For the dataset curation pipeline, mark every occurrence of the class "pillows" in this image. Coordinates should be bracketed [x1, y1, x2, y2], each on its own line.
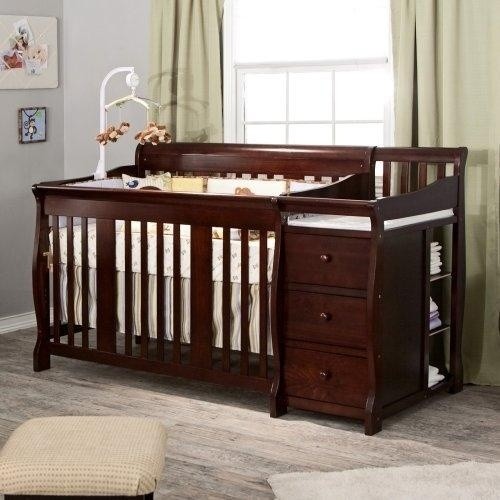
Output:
[204, 176, 286, 242]
[121, 172, 172, 235]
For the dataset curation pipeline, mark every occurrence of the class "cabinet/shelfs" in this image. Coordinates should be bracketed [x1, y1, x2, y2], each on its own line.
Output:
[282, 207, 457, 437]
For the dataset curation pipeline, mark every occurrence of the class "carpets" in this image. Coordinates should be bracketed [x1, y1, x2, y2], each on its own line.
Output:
[267, 463, 499, 500]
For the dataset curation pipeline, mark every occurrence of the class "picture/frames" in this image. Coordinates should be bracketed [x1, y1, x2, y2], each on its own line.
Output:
[18, 106, 46, 144]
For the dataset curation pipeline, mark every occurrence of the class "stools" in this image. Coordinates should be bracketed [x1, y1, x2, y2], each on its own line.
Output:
[0, 415, 166, 499]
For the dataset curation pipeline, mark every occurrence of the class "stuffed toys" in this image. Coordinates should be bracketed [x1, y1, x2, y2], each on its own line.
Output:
[95, 120, 172, 146]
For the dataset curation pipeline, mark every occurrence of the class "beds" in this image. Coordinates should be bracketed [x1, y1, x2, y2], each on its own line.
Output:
[28, 137, 375, 396]
